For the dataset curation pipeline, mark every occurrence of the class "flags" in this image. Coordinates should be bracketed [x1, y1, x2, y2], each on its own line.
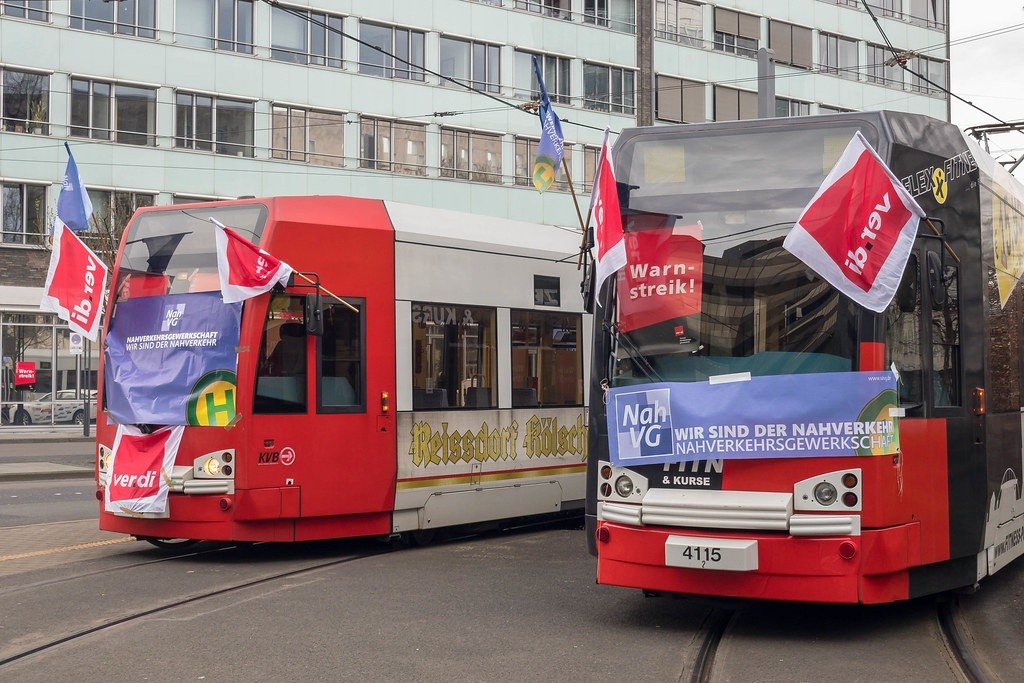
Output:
[214, 220, 292, 304]
[617, 210, 675, 314]
[784, 133, 925, 313]
[50, 154, 93, 234]
[533, 61, 565, 194]
[41, 219, 108, 341]
[594, 142, 627, 307]
[143, 234, 185, 274]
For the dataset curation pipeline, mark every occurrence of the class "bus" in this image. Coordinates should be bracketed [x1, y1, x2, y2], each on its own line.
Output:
[580, 110, 1024, 604]
[95, 195, 615, 550]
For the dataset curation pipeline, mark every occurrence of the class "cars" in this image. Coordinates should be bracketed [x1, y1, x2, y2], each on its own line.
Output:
[8, 388, 97, 426]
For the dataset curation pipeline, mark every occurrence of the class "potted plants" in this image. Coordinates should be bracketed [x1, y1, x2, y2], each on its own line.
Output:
[30, 99, 47, 134]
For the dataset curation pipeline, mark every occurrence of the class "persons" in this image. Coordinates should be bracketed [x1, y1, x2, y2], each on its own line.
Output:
[833, 314, 953, 408]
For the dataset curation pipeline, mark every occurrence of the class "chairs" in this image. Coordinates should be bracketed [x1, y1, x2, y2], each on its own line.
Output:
[254, 320, 543, 413]
